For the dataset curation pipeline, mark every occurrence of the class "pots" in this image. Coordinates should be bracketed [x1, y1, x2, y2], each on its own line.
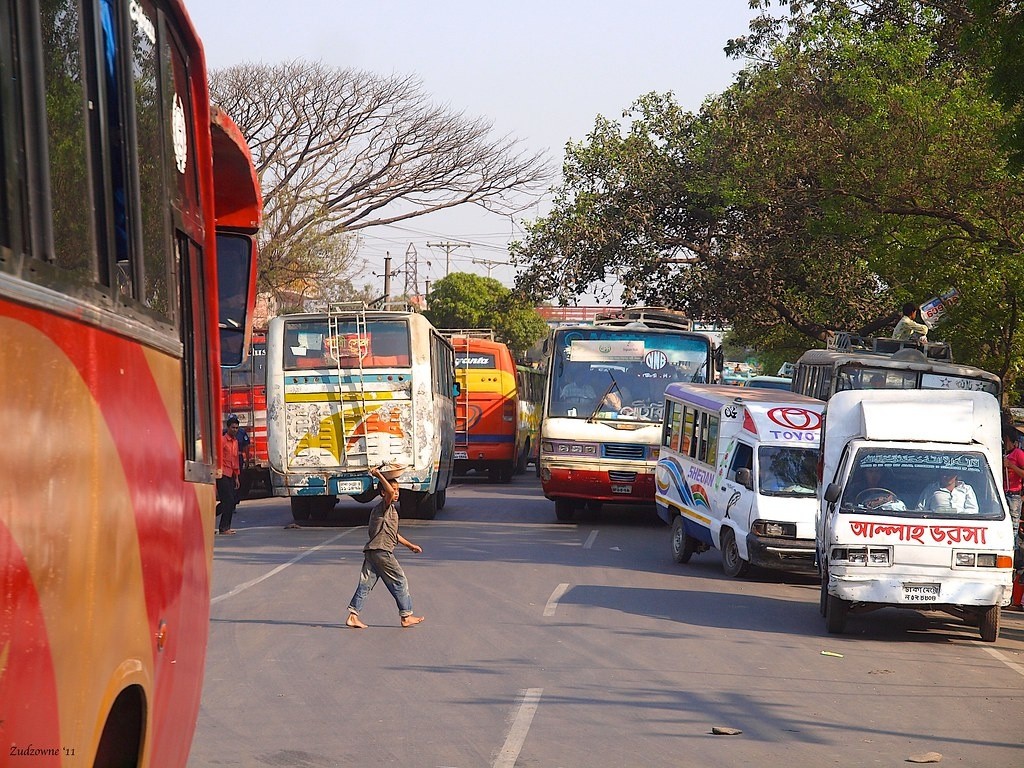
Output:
[371, 460, 407, 480]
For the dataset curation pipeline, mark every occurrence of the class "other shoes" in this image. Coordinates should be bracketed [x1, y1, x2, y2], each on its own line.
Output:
[219, 528, 237, 534]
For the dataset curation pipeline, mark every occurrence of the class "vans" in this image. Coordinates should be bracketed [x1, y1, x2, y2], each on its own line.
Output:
[720, 375, 793, 390]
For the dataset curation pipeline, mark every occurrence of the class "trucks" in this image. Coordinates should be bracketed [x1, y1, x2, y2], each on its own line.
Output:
[655, 382, 827, 578]
[814, 390, 1015, 642]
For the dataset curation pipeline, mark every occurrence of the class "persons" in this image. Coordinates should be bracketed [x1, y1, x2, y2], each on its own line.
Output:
[843, 459, 895, 505]
[891, 303, 929, 343]
[214, 413, 250, 535]
[559, 366, 675, 416]
[747, 451, 792, 490]
[916, 465, 979, 515]
[735, 363, 742, 372]
[346, 465, 425, 628]
[997, 424, 1024, 550]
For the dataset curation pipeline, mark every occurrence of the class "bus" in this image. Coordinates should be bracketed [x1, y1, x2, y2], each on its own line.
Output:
[436, 328, 522, 483]
[267, 301, 461, 522]
[1, 0, 261, 767]
[222, 329, 272, 498]
[541, 306, 725, 521]
[792, 331, 1002, 402]
[513, 357, 546, 477]
